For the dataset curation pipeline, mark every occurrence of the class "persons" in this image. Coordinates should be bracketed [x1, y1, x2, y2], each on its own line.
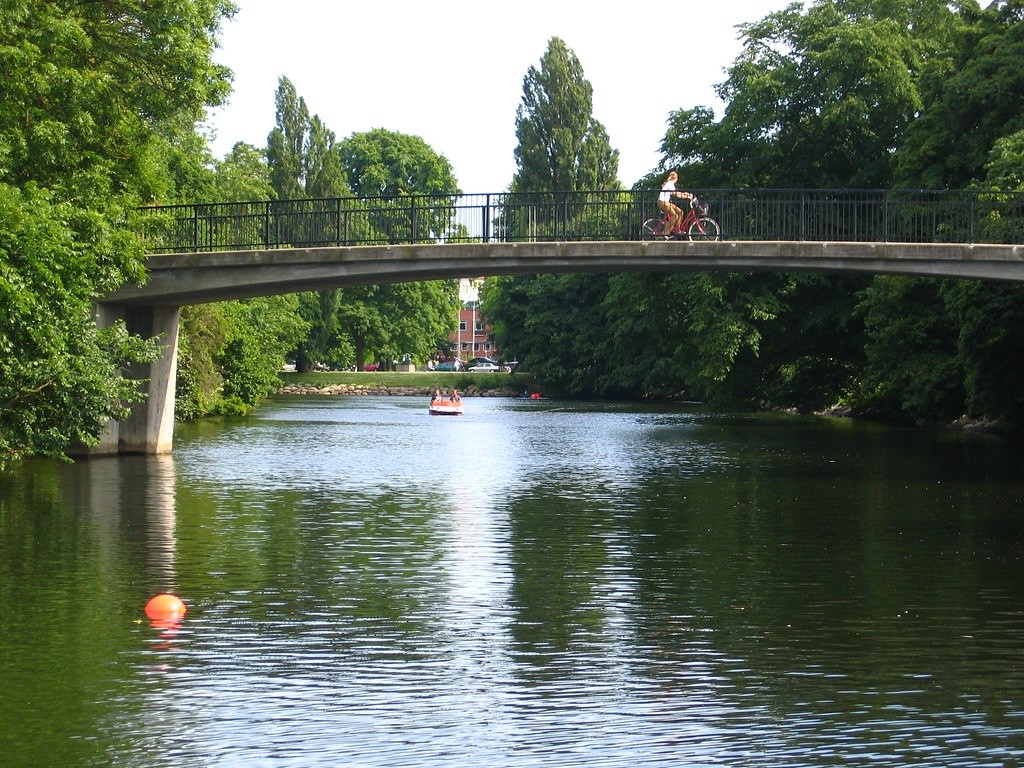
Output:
[657, 171, 693, 241]
[315, 360, 323, 371]
[431, 388, 443, 403]
[450, 388, 461, 402]
[453, 354, 464, 371]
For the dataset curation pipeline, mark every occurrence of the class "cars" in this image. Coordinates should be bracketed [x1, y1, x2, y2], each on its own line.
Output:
[315, 361, 330, 371]
[464, 357, 497, 370]
[283, 359, 296, 371]
[468, 363, 500, 372]
[436, 360, 465, 372]
[330, 363, 345, 371]
[362, 362, 381, 371]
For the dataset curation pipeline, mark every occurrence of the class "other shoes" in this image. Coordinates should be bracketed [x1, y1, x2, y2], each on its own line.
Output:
[662, 232, 669, 240]
[678, 231, 686, 235]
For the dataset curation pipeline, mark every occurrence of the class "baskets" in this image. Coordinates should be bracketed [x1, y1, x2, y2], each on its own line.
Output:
[698, 204, 709, 216]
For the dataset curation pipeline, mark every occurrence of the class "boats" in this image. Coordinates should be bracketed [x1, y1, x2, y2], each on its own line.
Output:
[517, 392, 551, 401]
[428, 395, 463, 415]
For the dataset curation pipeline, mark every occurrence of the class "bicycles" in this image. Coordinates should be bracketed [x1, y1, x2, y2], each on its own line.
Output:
[639, 195, 720, 242]
